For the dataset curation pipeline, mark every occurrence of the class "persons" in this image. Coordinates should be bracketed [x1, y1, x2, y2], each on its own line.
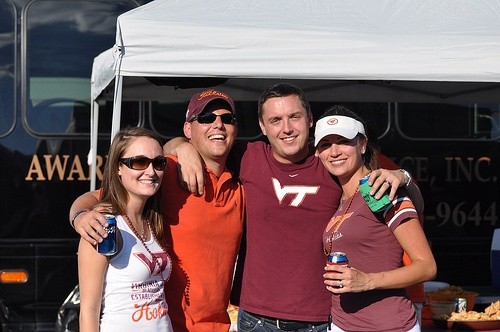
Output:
[163, 82, 424, 332]
[70, 90, 246, 332]
[78, 128, 172, 332]
[314, 107, 437, 332]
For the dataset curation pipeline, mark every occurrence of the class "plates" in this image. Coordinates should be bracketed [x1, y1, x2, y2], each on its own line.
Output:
[446, 318, 500, 329]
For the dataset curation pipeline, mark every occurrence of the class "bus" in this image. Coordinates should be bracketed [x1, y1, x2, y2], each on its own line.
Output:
[0, 1, 500, 332]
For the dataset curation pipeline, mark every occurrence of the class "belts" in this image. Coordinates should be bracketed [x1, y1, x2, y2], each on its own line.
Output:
[250, 314, 324, 330]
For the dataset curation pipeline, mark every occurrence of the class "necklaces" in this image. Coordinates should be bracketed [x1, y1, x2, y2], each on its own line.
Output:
[323, 187, 358, 256]
[338, 197, 348, 212]
[121, 208, 172, 283]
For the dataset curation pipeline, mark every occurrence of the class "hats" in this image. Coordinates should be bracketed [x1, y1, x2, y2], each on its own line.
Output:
[186, 88, 235, 122]
[314, 115, 366, 147]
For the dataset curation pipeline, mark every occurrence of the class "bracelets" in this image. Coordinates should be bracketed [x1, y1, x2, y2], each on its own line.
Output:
[71, 209, 90, 228]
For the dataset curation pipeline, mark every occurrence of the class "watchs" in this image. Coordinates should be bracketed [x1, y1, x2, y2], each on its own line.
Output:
[399, 169, 413, 186]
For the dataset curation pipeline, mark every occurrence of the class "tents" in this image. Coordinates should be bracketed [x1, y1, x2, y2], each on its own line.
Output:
[90, 0, 500, 191]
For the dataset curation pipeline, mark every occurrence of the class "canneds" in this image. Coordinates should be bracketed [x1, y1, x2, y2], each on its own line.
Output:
[328, 251, 348, 264]
[455, 298, 467, 312]
[359, 175, 370, 185]
[96, 214, 116, 256]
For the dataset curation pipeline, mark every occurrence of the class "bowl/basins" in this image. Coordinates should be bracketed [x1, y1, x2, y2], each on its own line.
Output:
[429, 300, 458, 317]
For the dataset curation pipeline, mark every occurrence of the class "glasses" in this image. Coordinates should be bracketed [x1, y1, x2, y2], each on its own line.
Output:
[121, 156, 167, 171]
[191, 112, 236, 124]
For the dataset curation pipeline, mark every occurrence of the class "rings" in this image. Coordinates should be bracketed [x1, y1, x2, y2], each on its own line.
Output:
[339, 281, 343, 287]
[385, 180, 390, 186]
